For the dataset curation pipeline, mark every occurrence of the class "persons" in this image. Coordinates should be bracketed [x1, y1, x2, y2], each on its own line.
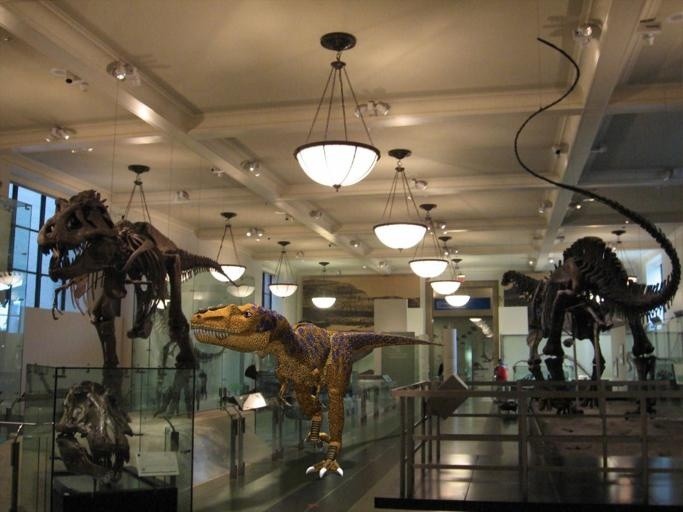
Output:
[494, 359, 507, 392]
[199, 370, 207, 400]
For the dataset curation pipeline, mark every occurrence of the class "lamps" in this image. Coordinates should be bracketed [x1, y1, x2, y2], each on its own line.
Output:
[205, 208, 337, 311]
[290, 33, 381, 194]
[372, 148, 470, 309]
